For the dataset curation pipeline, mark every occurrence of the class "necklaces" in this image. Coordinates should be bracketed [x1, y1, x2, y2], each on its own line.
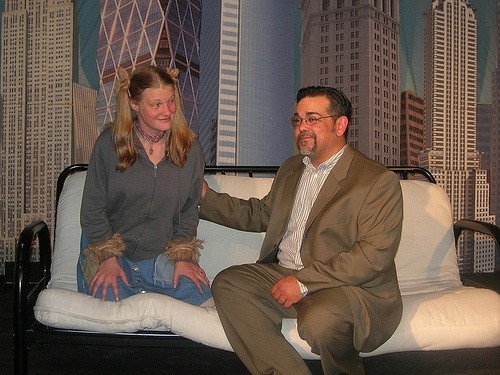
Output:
[136, 122, 166, 154]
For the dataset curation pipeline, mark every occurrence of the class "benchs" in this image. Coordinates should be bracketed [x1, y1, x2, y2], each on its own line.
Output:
[13, 164, 500, 375]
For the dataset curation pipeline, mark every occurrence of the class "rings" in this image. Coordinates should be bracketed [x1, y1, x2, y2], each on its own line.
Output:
[200, 272, 203, 275]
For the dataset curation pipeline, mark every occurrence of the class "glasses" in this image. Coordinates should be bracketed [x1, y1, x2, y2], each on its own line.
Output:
[291, 115, 341, 126]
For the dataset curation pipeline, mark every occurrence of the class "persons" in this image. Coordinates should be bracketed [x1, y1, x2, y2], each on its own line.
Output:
[200, 86, 404, 375]
[77, 65, 213, 306]
